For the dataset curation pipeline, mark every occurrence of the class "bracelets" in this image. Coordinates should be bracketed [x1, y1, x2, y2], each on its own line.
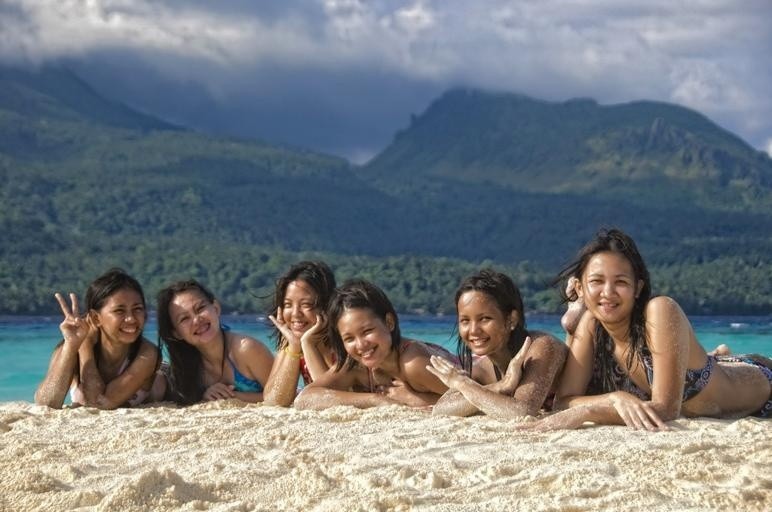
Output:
[284, 346, 303, 360]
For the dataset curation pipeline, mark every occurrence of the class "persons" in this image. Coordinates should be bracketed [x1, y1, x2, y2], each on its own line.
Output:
[157, 280, 301, 404]
[33, 267, 169, 410]
[519, 225, 772, 432]
[264, 259, 348, 408]
[295, 278, 480, 409]
[426, 268, 587, 422]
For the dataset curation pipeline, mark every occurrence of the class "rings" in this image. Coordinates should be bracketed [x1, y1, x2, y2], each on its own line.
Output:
[441, 369, 448, 373]
